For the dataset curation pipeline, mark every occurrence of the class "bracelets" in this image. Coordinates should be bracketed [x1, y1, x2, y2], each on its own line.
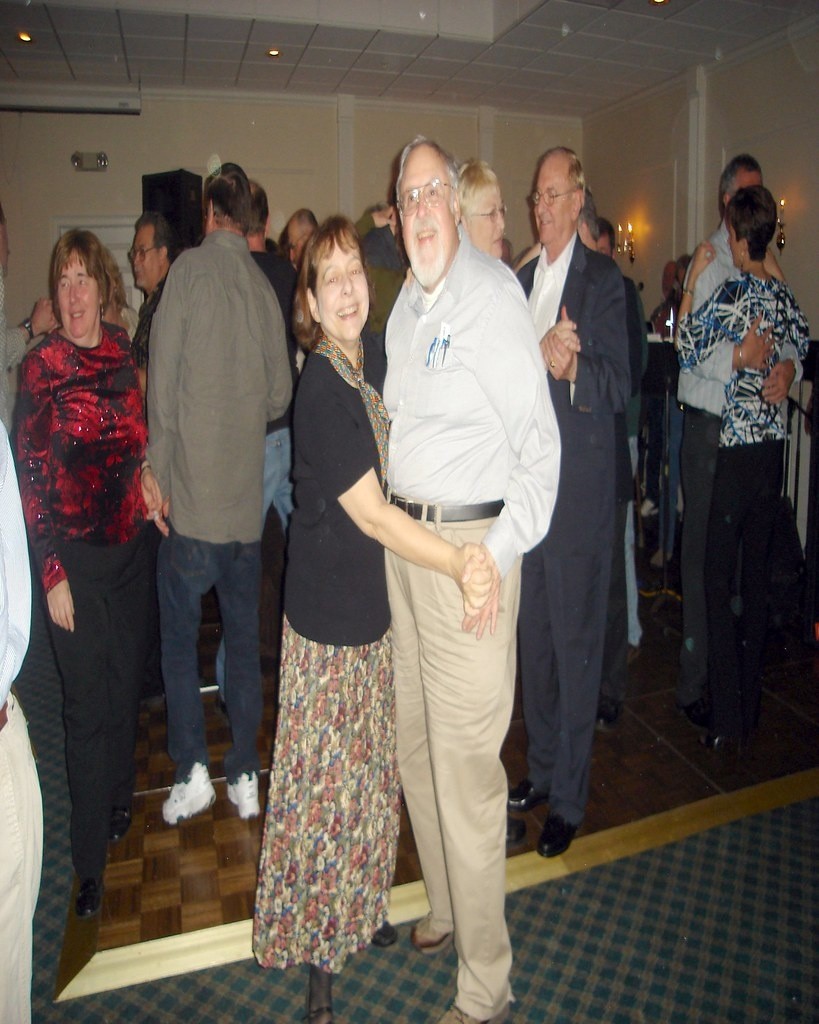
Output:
[142, 465, 151, 472]
[682, 289, 694, 296]
[738, 344, 743, 369]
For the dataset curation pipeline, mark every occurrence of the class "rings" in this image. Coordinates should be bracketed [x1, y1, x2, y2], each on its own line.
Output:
[550, 362, 556, 368]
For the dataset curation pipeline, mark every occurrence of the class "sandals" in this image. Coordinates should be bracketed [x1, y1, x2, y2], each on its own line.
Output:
[696, 732, 725, 750]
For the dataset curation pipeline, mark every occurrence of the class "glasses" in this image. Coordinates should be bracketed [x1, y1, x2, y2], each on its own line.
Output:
[288, 232, 308, 249]
[531, 189, 577, 206]
[395, 178, 455, 217]
[128, 245, 160, 262]
[470, 204, 507, 223]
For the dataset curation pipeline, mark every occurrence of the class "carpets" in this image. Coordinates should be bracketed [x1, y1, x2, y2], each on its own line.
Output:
[11, 560, 819, 1024]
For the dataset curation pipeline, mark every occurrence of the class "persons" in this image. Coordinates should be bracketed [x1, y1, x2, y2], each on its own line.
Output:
[15, 153, 810, 917]
[254, 213, 493, 1024]
[0, 204, 58, 1024]
[455, 156, 582, 846]
[376, 134, 560, 1024]
[507, 146, 632, 857]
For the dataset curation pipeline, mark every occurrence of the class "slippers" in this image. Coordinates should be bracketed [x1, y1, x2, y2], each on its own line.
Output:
[304, 985, 333, 1024]
[372, 920, 399, 947]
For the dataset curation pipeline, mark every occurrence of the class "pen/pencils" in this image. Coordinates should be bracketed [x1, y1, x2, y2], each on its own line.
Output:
[426, 337, 442, 367]
[441, 335, 451, 365]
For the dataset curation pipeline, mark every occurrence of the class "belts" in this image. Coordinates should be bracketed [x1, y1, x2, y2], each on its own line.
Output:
[383, 478, 505, 524]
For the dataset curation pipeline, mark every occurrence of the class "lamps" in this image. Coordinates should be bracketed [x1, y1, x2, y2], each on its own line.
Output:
[616, 222, 636, 264]
[72, 151, 109, 173]
[776, 197, 787, 256]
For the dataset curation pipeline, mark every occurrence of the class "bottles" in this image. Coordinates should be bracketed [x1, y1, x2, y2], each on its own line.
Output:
[664, 303, 677, 342]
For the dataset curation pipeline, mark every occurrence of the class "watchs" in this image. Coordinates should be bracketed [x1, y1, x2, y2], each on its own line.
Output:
[23, 320, 34, 339]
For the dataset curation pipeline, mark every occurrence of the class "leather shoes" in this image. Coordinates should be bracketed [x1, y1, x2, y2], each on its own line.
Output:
[595, 696, 625, 729]
[506, 814, 527, 844]
[506, 778, 551, 811]
[675, 695, 713, 728]
[109, 800, 132, 843]
[74, 873, 105, 918]
[537, 812, 578, 858]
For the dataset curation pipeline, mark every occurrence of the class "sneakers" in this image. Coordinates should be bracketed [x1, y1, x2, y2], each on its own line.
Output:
[226, 769, 260, 820]
[161, 761, 216, 826]
[438, 999, 510, 1024]
[667, 570, 684, 601]
[638, 561, 667, 597]
[411, 910, 455, 955]
